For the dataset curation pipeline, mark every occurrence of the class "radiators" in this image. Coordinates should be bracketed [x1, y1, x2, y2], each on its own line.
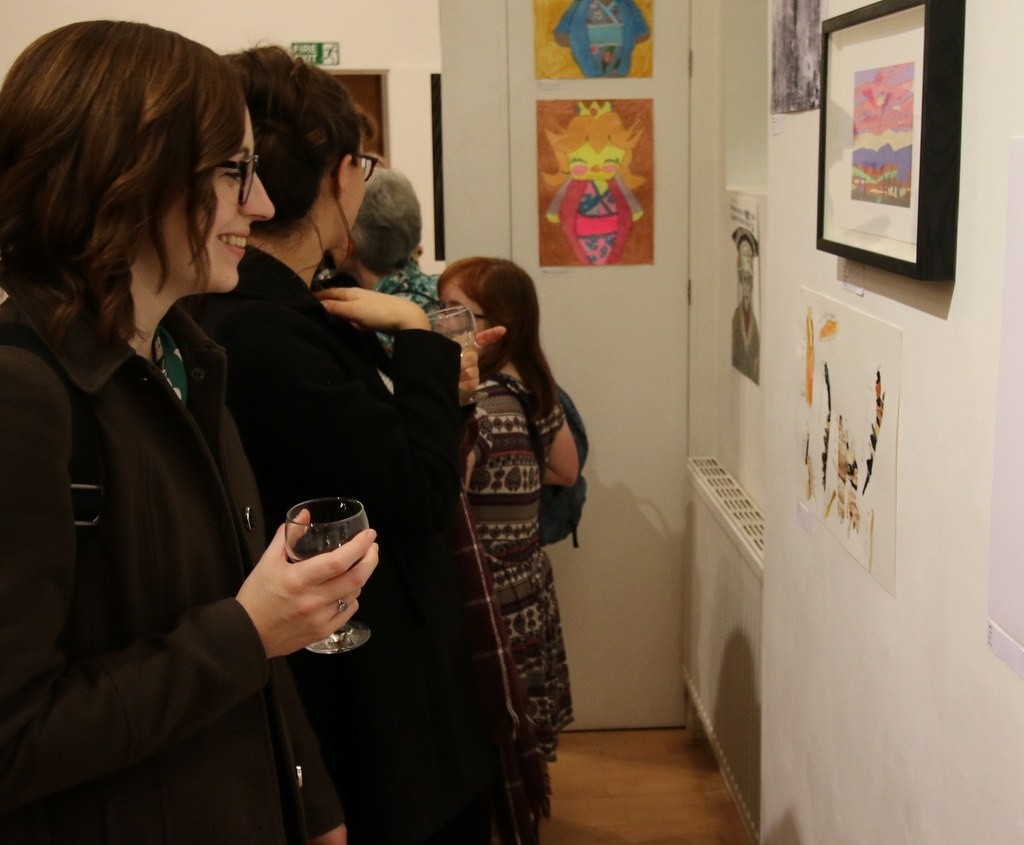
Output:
[684, 456, 764, 845]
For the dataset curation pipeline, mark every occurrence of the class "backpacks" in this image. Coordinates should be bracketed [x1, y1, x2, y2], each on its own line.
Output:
[488, 373, 589, 548]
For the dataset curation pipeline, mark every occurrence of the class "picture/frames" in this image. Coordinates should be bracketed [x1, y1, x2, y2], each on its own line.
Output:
[817, 0, 965, 279]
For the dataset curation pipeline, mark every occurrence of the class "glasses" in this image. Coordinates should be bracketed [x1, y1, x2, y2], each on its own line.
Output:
[213, 154, 260, 206]
[436, 303, 488, 320]
[357, 153, 379, 182]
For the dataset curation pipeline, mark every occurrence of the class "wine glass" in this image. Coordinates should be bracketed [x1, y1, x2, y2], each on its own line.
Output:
[284, 495, 371, 655]
[427, 304, 489, 407]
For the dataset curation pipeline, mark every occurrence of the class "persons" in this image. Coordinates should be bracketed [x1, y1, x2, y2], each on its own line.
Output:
[182, 46, 508, 845]
[324, 168, 581, 832]
[731, 227, 760, 386]
[0, 21, 380, 845]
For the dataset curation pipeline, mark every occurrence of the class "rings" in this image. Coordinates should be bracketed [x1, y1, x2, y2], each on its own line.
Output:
[469, 331, 483, 350]
[338, 599, 348, 612]
